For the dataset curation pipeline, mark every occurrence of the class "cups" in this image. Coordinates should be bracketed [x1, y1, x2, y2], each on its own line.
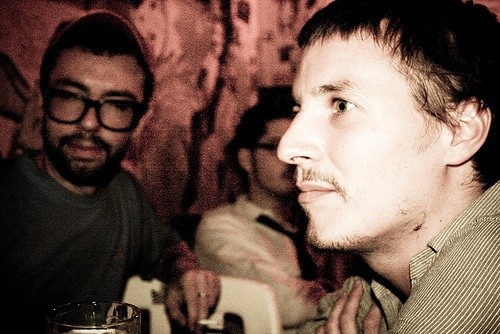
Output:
[47, 300, 141, 334]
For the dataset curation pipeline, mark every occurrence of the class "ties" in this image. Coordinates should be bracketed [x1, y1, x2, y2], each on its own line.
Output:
[257, 216, 320, 282]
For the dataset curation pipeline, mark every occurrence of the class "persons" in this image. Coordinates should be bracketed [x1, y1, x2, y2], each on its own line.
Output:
[277, 0, 500, 334]
[0, 8, 356, 334]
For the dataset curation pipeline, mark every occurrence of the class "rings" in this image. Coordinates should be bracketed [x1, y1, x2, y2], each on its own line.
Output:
[197, 291, 210, 300]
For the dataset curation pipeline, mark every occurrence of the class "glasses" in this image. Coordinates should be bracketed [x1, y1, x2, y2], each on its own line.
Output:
[256, 141, 279, 156]
[44, 88, 141, 133]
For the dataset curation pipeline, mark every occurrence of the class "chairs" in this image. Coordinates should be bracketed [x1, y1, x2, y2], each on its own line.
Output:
[123, 271, 284, 334]
